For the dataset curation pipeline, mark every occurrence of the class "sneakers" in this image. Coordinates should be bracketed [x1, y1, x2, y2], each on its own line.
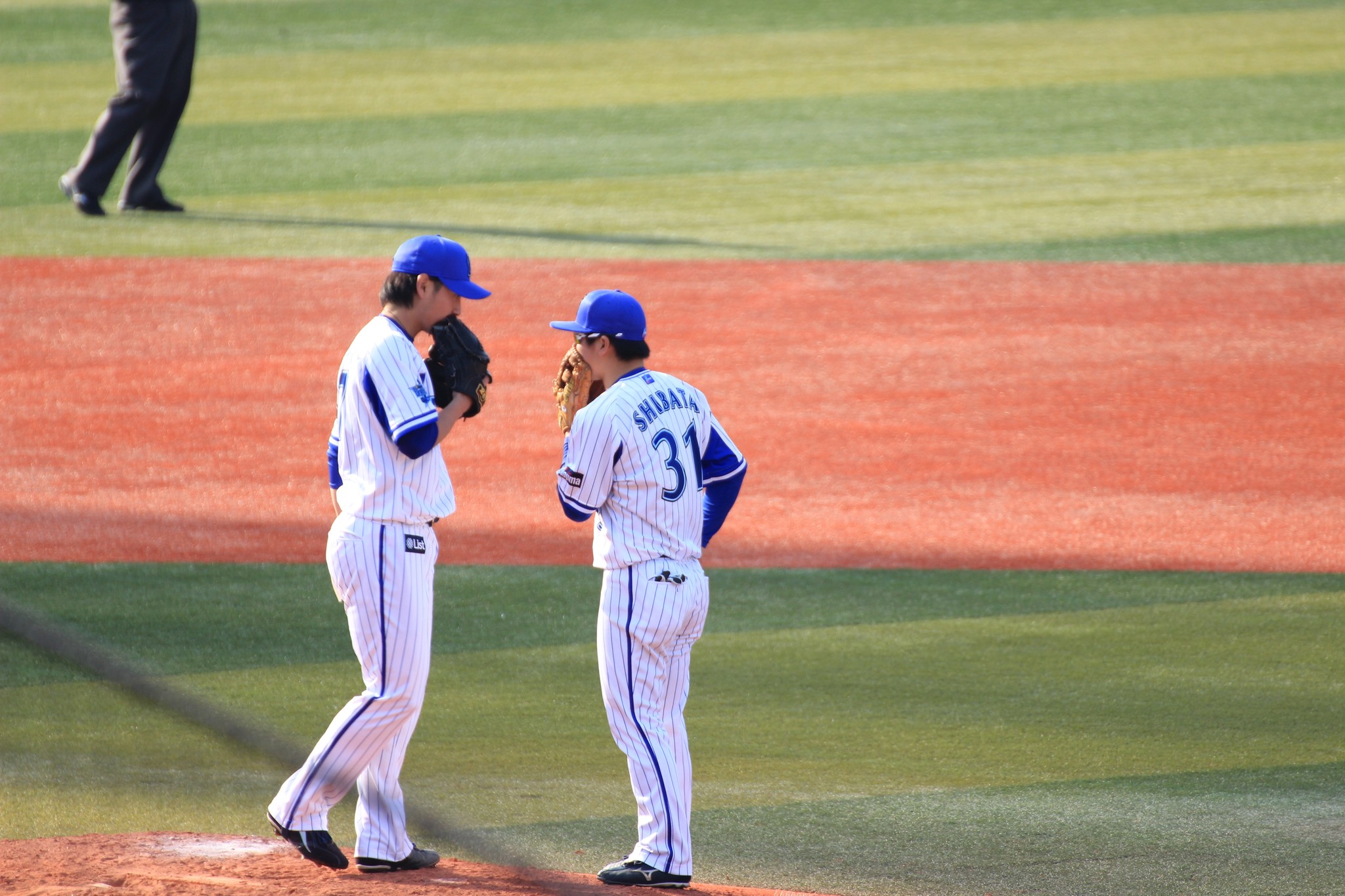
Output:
[356, 843, 442, 872]
[266, 811, 349, 871]
[598, 855, 691, 887]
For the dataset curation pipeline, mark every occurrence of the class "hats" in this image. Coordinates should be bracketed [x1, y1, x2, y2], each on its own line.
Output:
[549, 289, 648, 340]
[392, 233, 493, 299]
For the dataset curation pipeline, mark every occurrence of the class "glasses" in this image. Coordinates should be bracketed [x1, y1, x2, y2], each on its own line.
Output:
[574, 333, 600, 343]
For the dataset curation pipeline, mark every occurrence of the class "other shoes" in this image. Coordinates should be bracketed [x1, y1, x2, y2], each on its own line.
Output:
[58, 172, 105, 215]
[119, 194, 183, 212]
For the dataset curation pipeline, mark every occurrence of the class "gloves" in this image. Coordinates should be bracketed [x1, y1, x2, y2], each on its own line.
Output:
[655, 570, 687, 584]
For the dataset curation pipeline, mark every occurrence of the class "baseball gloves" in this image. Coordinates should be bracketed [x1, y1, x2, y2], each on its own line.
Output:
[552, 341, 606, 434]
[423, 312, 492, 418]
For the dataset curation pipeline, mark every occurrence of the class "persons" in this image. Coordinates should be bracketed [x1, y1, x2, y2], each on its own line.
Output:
[56, 0, 201, 216]
[266, 235, 492, 872]
[549, 290, 749, 888]
